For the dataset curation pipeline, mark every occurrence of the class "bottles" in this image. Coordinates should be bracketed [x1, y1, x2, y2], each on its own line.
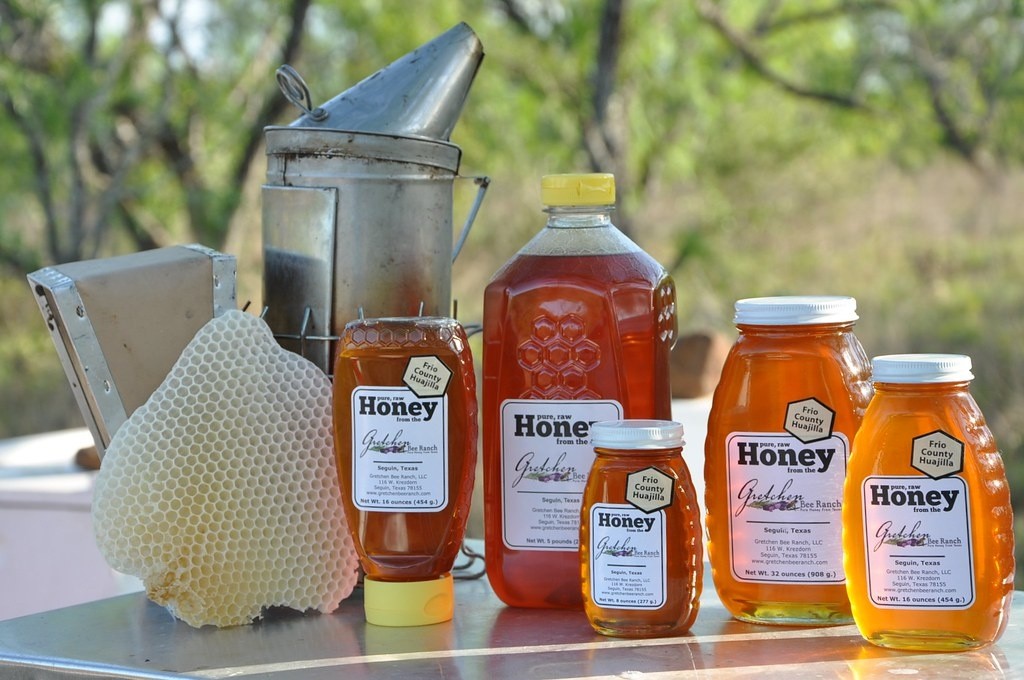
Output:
[704, 297, 881, 624]
[325, 317, 476, 626]
[576, 418, 705, 639]
[479, 175, 678, 602]
[840, 350, 1016, 654]
